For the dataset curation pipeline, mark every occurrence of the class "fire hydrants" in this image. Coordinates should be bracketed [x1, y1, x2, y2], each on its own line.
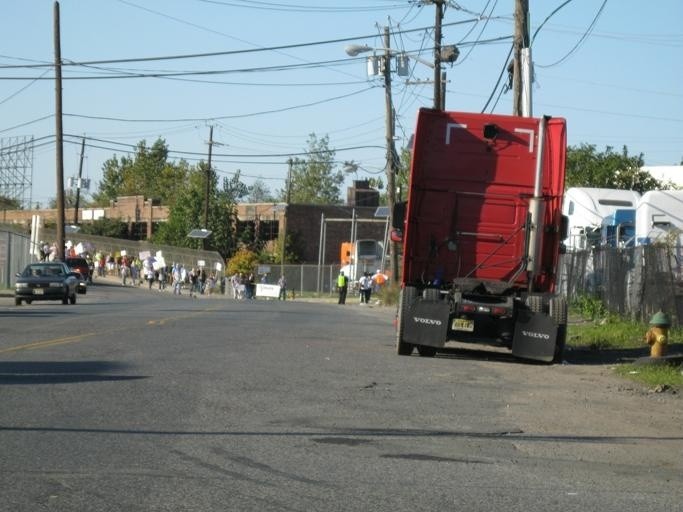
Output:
[643, 306, 672, 357]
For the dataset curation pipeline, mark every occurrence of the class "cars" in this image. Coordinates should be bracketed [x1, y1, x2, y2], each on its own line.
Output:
[13, 261, 79, 305]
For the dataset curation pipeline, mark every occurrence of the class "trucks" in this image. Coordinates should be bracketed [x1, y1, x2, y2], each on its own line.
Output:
[395, 107, 571, 366]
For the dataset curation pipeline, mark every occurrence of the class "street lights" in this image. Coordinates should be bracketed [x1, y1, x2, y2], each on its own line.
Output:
[345, 0, 460, 111]
[342, 162, 387, 177]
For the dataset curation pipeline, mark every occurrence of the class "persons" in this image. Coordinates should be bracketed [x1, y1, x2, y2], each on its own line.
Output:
[261, 273, 268, 284]
[278, 274, 287, 300]
[335, 271, 348, 304]
[39, 242, 254, 300]
[358, 269, 389, 306]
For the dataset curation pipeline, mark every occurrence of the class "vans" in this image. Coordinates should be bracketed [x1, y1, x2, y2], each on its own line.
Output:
[66, 256, 91, 284]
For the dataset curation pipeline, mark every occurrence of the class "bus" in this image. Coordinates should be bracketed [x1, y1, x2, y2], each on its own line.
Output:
[353, 240, 385, 287]
